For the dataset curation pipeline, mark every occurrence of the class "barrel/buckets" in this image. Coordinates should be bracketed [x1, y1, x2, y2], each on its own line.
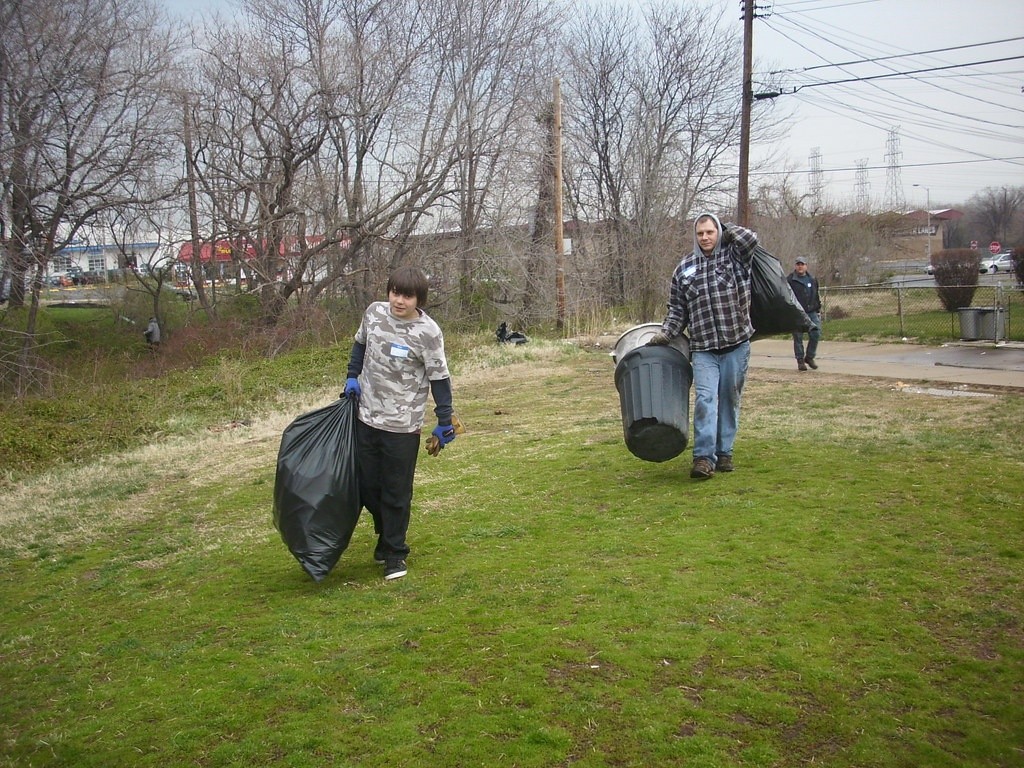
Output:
[613, 322, 690, 366]
[614, 344, 696, 461]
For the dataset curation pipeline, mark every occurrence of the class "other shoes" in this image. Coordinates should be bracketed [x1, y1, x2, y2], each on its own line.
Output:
[798, 358, 807, 371]
[805, 356, 817, 369]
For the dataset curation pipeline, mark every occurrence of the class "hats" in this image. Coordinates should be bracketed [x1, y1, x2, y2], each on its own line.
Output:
[796, 257, 806, 264]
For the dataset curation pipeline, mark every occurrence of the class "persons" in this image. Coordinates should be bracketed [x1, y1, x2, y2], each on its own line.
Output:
[650, 213, 759, 477]
[143, 316, 161, 346]
[344, 265, 455, 581]
[785, 257, 820, 369]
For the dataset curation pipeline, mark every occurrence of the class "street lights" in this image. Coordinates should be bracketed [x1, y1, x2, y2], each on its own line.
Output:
[913, 183, 931, 265]
[1002, 186, 1005, 253]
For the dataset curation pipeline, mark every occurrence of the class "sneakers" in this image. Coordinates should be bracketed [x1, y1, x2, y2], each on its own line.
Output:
[690, 459, 714, 478]
[717, 455, 734, 471]
[374, 544, 387, 564]
[384, 558, 407, 581]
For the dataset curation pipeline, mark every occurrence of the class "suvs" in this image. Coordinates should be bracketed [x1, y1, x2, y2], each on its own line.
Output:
[978, 253, 1014, 274]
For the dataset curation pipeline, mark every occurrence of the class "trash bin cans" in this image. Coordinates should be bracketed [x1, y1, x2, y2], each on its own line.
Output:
[957, 307, 981, 339]
[981, 307, 1006, 340]
[613, 322, 693, 463]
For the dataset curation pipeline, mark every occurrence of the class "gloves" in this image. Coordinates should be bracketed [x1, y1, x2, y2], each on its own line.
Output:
[645, 332, 670, 345]
[426, 415, 466, 457]
[432, 425, 455, 449]
[344, 378, 361, 398]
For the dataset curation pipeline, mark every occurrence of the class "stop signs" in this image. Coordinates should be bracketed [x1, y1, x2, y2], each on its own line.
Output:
[971, 241, 977, 249]
[989, 242, 1001, 254]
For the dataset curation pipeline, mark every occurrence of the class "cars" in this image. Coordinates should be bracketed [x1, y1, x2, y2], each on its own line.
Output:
[84, 266, 185, 283]
[3, 267, 85, 300]
[1005, 248, 1014, 253]
[924, 264, 936, 274]
